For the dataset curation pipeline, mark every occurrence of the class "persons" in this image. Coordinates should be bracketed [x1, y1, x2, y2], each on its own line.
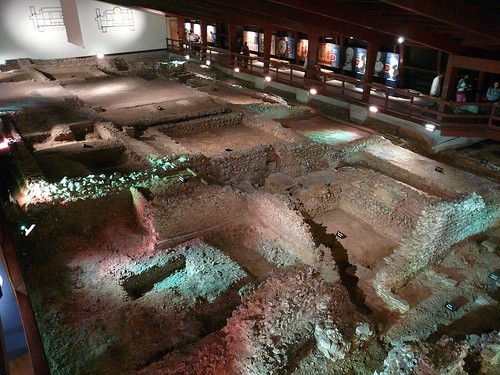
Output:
[453, 72, 473, 113]
[210, 31, 216, 42]
[177, 28, 200, 55]
[487, 81, 500, 116]
[428, 70, 444, 110]
[243, 41, 250, 68]
